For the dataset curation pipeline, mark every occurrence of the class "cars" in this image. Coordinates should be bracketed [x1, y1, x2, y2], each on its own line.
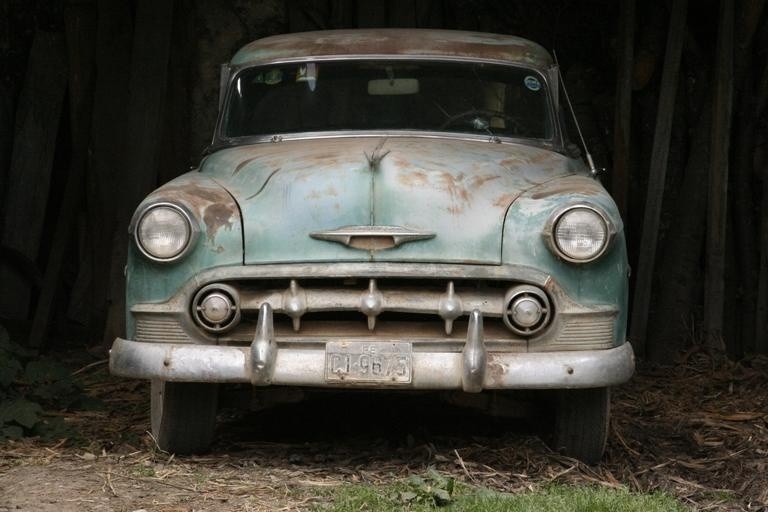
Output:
[109, 25, 637, 466]
